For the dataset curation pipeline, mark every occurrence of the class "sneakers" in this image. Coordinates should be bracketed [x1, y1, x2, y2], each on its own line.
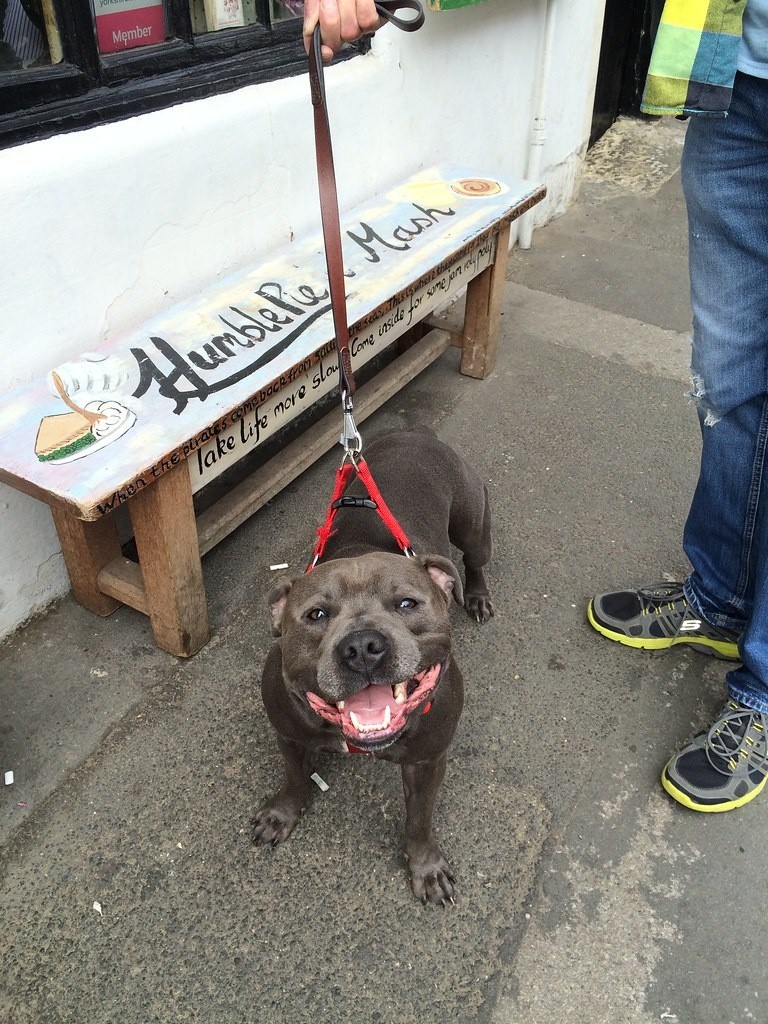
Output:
[588, 582, 742, 661]
[661, 695, 768, 813]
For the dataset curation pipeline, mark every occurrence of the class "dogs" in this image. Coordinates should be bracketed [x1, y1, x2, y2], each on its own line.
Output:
[247, 432, 491, 911]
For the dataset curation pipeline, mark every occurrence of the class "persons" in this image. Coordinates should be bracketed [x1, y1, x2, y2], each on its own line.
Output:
[303, 0, 768, 813]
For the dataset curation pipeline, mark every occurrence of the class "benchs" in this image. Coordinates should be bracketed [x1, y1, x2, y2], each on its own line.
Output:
[0, 155, 547, 656]
